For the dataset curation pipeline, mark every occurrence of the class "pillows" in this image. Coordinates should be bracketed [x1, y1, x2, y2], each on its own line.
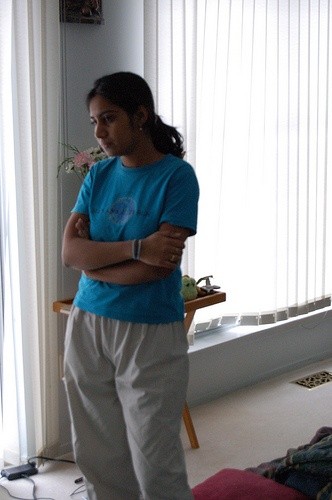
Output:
[192, 468, 307, 500]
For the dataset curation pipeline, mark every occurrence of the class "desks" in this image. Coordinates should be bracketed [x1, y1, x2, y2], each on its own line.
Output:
[52, 286, 227, 451]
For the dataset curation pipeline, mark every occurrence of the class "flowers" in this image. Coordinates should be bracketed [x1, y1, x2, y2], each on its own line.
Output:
[55, 141, 108, 183]
